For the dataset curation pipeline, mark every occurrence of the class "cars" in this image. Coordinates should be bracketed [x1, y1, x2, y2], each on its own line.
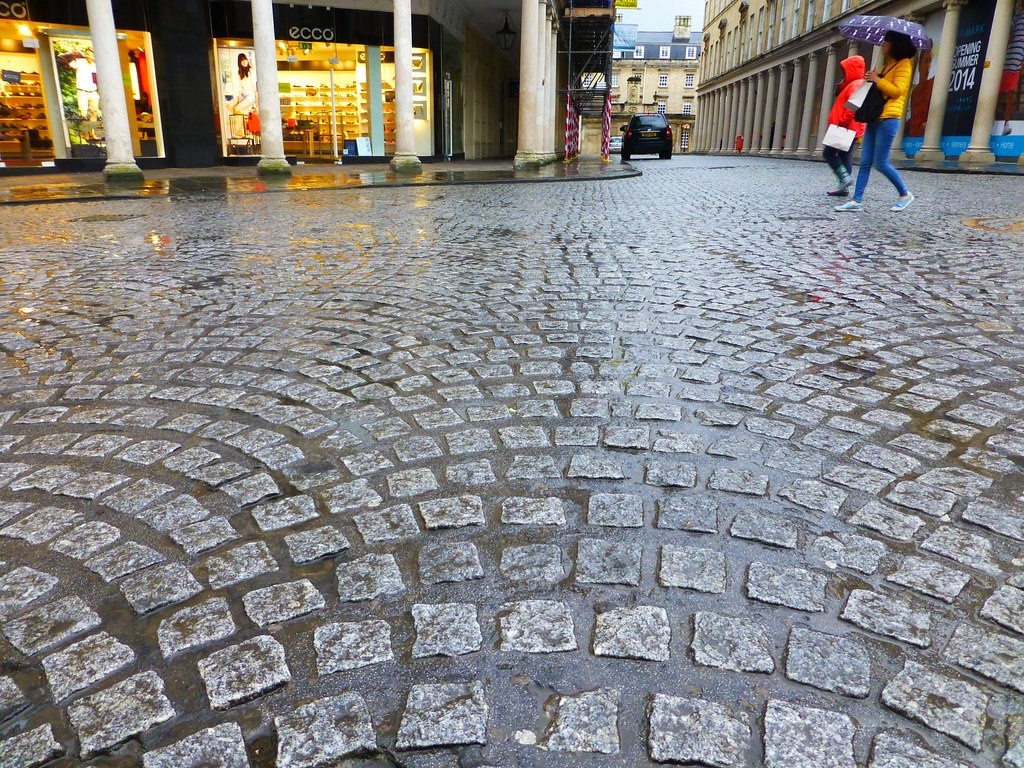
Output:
[609, 137, 622, 154]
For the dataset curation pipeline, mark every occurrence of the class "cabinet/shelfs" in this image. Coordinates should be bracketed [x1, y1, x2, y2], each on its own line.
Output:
[0, 71, 55, 160]
[292, 82, 396, 156]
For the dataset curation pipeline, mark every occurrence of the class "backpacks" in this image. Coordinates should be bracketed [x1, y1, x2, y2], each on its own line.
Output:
[854, 74, 884, 122]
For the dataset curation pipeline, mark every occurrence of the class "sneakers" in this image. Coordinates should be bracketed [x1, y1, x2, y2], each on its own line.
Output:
[834, 202, 864, 212]
[890, 191, 914, 211]
[826, 189, 849, 195]
[842, 177, 854, 188]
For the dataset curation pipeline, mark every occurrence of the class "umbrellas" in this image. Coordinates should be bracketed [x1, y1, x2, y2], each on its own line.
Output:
[838, 15, 930, 82]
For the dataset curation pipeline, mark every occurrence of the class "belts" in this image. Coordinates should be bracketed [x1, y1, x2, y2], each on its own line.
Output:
[78, 88, 97, 93]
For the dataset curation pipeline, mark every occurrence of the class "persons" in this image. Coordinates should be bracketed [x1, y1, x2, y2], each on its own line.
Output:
[822, 55, 866, 196]
[226, 53, 257, 116]
[68, 47, 101, 140]
[907, 39, 935, 137]
[834, 30, 916, 211]
[989, 0, 1024, 136]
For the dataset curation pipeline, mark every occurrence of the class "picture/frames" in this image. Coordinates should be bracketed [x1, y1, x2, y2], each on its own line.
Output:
[412, 77, 426, 95]
[414, 100, 428, 119]
[412, 52, 426, 71]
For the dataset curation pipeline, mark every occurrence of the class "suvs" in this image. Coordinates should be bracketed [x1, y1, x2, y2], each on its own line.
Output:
[619, 112, 673, 160]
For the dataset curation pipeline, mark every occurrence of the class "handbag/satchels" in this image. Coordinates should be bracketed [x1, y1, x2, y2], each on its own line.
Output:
[845, 80, 872, 111]
[822, 122, 857, 152]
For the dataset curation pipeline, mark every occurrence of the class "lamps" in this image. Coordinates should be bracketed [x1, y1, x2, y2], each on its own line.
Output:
[495, 9, 516, 50]
